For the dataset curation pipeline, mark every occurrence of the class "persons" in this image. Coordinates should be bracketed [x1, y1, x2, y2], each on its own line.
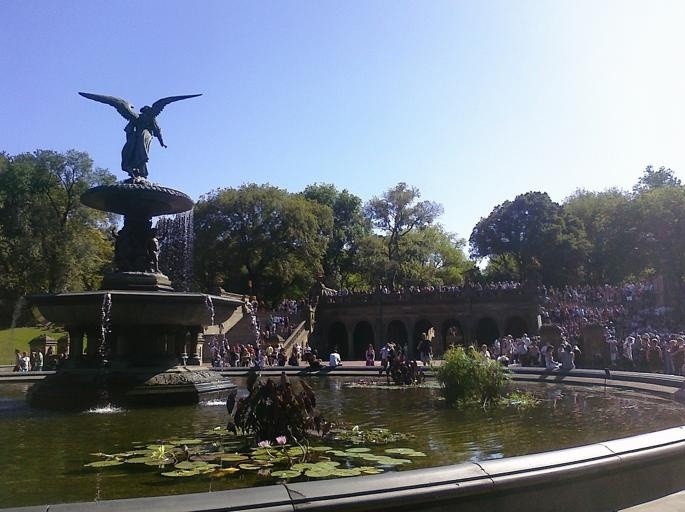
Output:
[415, 330, 436, 366]
[120, 106, 167, 178]
[13, 345, 68, 371]
[365, 340, 408, 366]
[208, 289, 345, 368]
[465, 279, 685, 377]
[343, 284, 459, 293]
